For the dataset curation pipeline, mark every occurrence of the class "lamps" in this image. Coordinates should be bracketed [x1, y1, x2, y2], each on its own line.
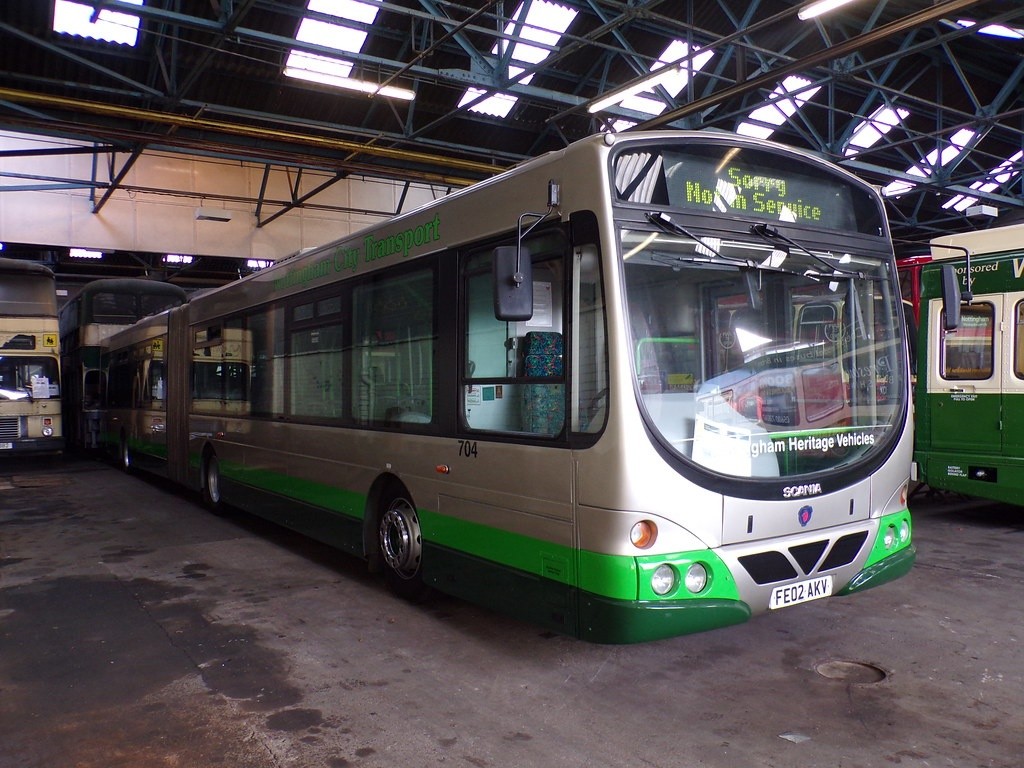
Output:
[195, 198, 232, 222]
[964, 200, 998, 218]
[585, 63, 680, 114]
[797, 0, 855, 21]
[280, 67, 417, 101]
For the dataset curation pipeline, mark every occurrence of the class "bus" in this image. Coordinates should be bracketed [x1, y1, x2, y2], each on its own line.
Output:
[714, 219, 1023, 506]
[58, 277, 189, 440]
[105, 129, 972, 646]
[101, 327, 254, 441]
[0, 257, 65, 455]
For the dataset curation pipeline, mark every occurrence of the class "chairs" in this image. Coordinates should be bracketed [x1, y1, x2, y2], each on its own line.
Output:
[519, 330, 584, 431]
